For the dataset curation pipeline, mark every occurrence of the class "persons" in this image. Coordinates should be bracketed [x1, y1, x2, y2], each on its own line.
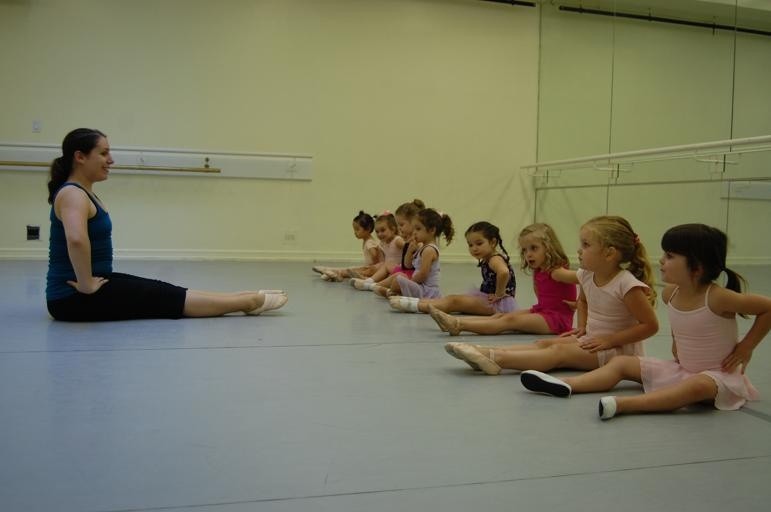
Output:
[429, 223, 582, 336]
[310, 198, 457, 302]
[444, 212, 661, 376]
[45, 126, 289, 318]
[521, 221, 771, 421]
[390, 220, 518, 318]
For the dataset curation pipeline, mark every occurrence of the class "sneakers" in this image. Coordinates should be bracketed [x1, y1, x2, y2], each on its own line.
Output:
[245, 290, 288, 315]
[598, 395, 617, 420]
[350, 276, 419, 312]
[427, 302, 461, 336]
[312, 266, 342, 282]
[444, 342, 502, 375]
[520, 369, 572, 398]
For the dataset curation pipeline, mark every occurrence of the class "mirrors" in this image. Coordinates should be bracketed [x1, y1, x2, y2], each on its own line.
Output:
[536, 0, 771, 267]
